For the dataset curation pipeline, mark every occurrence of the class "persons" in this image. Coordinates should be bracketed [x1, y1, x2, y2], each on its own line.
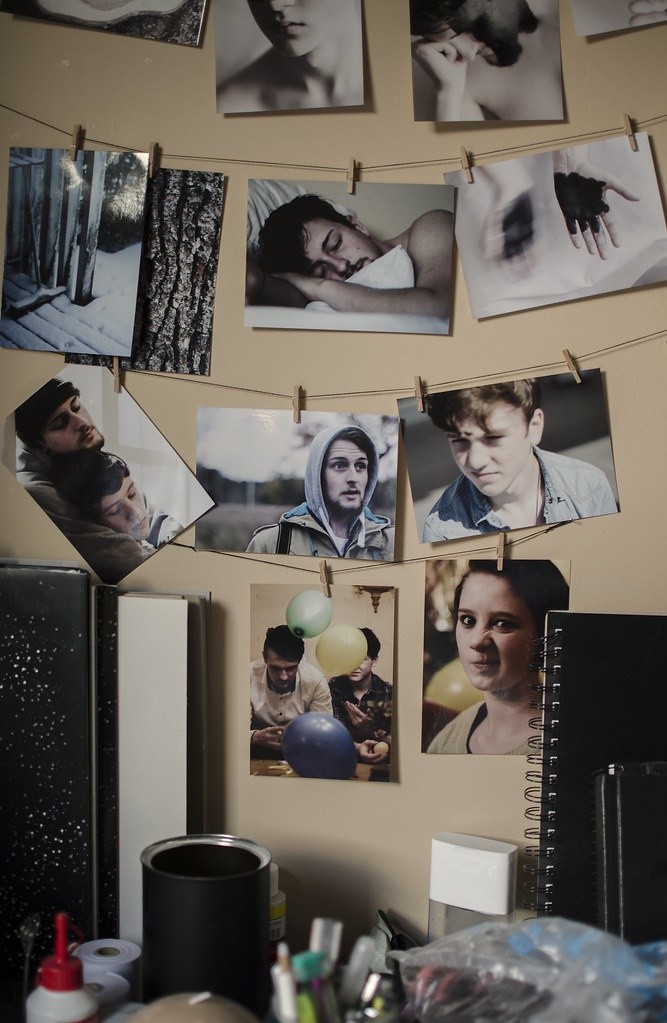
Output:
[241, 192, 452, 333]
[248, 620, 393, 764]
[624, 2, 666, 27]
[459, 143, 647, 292]
[422, 560, 571, 760]
[214, 0, 364, 115]
[415, 376, 621, 545]
[401, 0, 567, 126]
[6, 376, 187, 587]
[247, 421, 396, 567]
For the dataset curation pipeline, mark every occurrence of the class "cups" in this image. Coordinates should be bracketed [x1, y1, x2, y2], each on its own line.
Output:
[139, 833, 272, 1019]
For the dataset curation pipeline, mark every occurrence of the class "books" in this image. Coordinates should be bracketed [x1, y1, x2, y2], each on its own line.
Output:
[527, 607, 666, 956]
[0, 566, 214, 1023]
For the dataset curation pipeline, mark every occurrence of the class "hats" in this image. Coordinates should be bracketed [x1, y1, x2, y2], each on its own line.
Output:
[16, 376, 81, 447]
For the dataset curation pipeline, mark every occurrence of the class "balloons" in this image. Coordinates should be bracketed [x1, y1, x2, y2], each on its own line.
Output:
[285, 589, 332, 638]
[281, 712, 357, 781]
[315, 624, 368, 674]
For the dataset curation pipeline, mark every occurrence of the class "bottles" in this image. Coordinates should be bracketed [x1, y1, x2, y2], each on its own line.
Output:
[269, 863, 288, 969]
[25, 912, 100, 1023]
